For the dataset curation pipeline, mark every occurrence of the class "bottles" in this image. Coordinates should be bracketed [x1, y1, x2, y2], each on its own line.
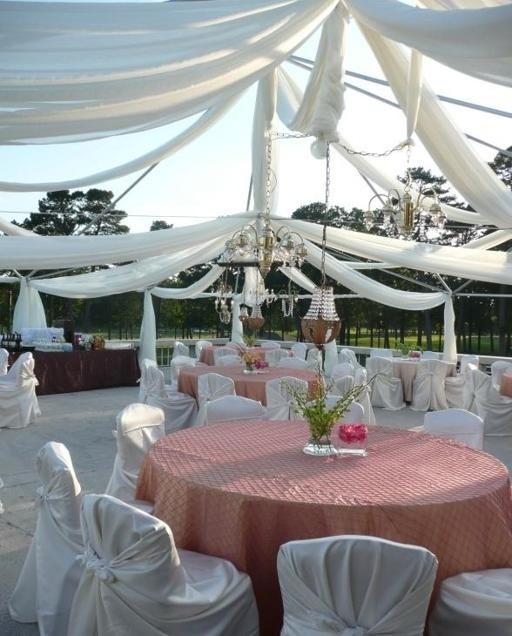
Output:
[1, 332, 22, 353]
[76, 335, 106, 351]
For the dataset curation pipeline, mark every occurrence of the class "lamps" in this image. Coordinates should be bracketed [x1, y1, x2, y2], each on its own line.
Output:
[363, 143, 447, 245]
[301, 137, 344, 349]
[205, 130, 311, 330]
